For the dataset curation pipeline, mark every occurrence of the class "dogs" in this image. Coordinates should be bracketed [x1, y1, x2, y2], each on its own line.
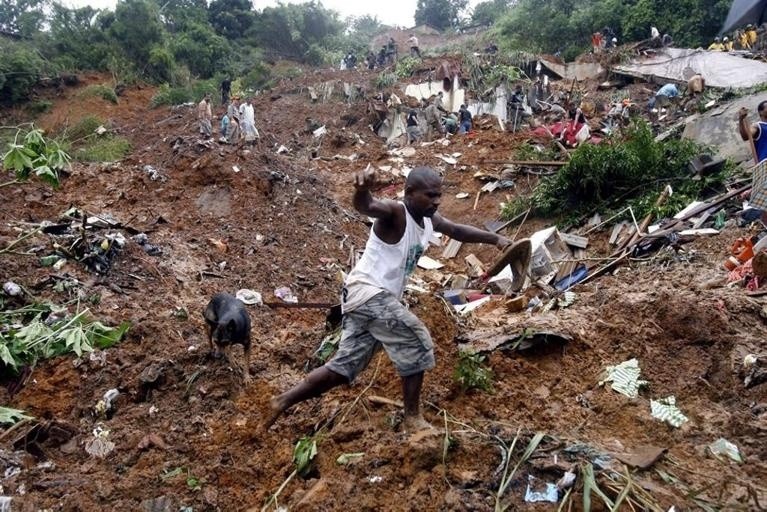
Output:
[202, 291, 253, 386]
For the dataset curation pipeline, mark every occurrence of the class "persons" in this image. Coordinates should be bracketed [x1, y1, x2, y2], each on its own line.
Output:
[238, 96, 266, 154]
[650, 25, 659, 39]
[262, 165, 515, 433]
[406, 91, 476, 145]
[340, 33, 423, 70]
[217, 79, 233, 105]
[226, 96, 240, 139]
[561, 102, 592, 139]
[198, 92, 214, 140]
[738, 100, 767, 227]
[707, 24, 758, 52]
[507, 85, 538, 133]
[221, 112, 229, 137]
